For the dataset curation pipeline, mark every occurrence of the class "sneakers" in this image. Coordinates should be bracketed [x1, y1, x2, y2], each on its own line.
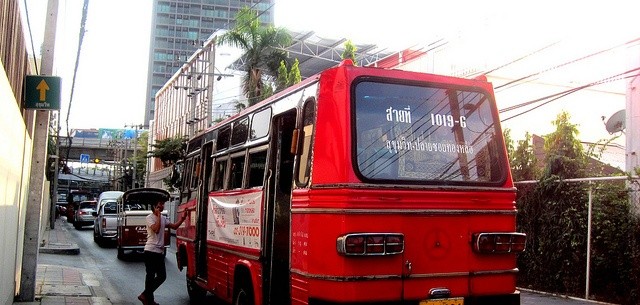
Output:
[138, 293, 159, 305]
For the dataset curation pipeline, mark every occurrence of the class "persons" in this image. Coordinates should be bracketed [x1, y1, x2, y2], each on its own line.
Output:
[136, 196, 189, 305]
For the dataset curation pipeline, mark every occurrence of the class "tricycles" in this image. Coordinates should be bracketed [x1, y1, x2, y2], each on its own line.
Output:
[115, 188, 172, 259]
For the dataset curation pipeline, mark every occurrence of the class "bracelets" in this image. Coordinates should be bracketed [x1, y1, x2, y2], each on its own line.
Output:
[180, 216, 185, 220]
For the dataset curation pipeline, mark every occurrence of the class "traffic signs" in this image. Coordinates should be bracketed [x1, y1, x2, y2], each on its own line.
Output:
[25, 75, 60, 110]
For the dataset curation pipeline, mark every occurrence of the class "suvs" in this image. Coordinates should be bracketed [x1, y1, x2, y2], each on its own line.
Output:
[73, 200, 96, 229]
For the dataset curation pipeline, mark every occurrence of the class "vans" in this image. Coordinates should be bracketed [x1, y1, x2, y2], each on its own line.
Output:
[96, 191, 125, 215]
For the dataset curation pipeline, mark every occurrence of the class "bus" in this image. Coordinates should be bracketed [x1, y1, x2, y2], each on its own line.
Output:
[176, 59, 528, 305]
[66, 190, 95, 222]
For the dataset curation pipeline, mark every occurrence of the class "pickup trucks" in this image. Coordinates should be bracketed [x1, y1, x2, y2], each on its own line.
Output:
[92, 200, 118, 242]
